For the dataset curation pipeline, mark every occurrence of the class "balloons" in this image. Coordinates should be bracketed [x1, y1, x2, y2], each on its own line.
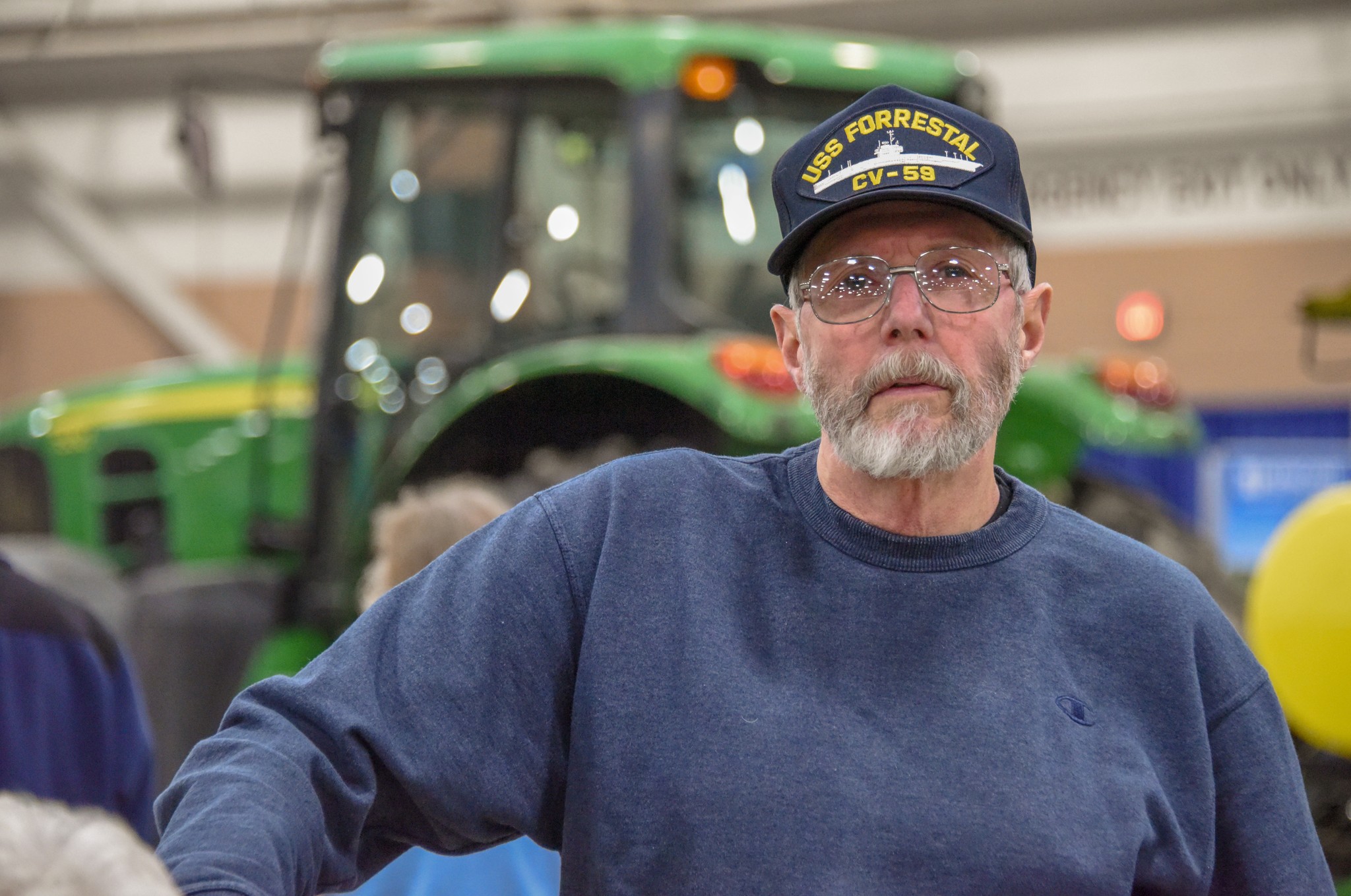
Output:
[1243, 484, 1350, 757]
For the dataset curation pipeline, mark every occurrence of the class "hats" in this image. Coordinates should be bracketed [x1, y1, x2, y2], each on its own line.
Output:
[767, 86, 1037, 295]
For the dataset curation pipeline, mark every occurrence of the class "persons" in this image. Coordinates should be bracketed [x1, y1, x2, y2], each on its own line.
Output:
[330, 475, 561, 896]
[154, 86, 1338, 896]
[0, 789, 186, 896]
[0, 545, 158, 847]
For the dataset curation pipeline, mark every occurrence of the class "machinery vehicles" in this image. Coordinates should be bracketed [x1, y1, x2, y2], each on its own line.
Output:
[0, 11, 1230, 706]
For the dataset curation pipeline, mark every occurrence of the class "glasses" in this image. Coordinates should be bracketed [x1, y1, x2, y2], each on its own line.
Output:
[793, 245, 1013, 324]
